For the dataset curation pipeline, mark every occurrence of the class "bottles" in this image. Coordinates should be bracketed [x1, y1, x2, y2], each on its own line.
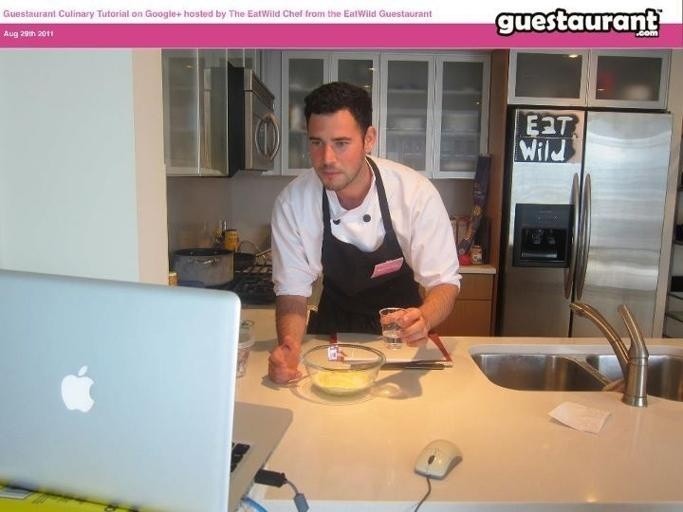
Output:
[449, 214, 468, 246]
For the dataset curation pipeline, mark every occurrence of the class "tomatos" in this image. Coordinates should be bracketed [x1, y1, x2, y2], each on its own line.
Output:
[459, 254, 472, 266]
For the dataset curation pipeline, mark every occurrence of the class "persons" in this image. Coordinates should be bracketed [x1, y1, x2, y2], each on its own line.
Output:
[268, 82, 463, 385]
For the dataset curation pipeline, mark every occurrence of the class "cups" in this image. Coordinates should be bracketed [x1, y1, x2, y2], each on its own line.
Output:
[379, 307, 409, 350]
[236, 328, 257, 379]
[223, 228, 240, 251]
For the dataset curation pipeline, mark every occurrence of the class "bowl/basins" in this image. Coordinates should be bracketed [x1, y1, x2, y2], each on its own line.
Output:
[302, 342, 387, 394]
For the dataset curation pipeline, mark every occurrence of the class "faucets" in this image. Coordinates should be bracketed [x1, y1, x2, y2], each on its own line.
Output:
[568, 300, 650, 409]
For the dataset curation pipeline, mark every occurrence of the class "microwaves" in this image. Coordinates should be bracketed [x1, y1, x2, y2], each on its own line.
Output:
[224, 63, 281, 176]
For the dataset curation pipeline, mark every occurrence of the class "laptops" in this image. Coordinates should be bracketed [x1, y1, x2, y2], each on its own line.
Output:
[0, 270, 293, 512]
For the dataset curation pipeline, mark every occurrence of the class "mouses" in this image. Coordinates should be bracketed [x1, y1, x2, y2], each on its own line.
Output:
[415, 439, 463, 480]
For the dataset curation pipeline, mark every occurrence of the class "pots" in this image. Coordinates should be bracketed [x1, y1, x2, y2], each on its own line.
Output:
[171, 247, 235, 285]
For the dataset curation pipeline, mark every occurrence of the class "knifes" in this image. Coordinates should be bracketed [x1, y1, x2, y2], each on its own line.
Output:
[349, 360, 445, 371]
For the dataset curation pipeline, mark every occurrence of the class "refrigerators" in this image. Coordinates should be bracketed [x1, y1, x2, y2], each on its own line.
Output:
[494, 105, 673, 340]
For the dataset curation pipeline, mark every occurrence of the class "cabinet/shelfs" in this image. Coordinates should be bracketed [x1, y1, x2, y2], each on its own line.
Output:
[227, 49, 268, 86]
[278, 49, 379, 177]
[161, 47, 229, 182]
[378, 51, 495, 180]
[417, 270, 496, 337]
[489, 48, 674, 111]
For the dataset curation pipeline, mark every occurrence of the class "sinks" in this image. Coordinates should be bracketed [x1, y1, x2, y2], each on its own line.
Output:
[586, 351, 683, 403]
[473, 351, 608, 391]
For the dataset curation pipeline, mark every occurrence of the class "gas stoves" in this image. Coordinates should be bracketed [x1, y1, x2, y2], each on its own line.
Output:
[199, 263, 278, 306]
